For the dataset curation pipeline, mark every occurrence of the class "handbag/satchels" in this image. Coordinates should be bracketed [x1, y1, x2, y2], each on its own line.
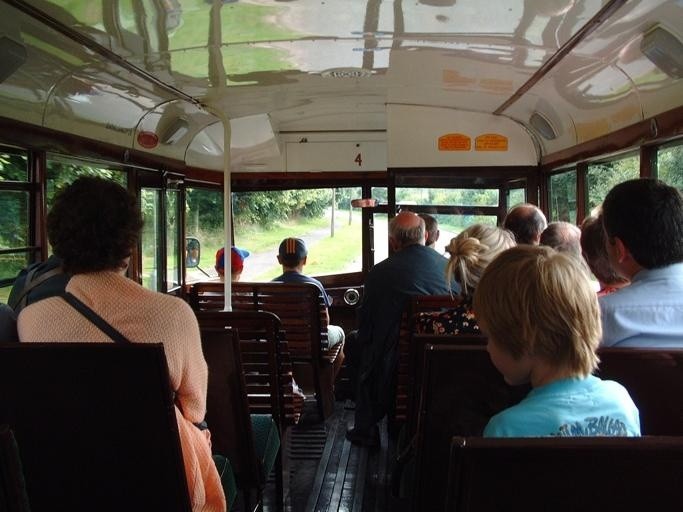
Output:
[172, 391, 208, 430]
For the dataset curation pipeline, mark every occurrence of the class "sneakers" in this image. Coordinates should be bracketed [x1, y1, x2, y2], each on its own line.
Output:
[345, 425, 381, 452]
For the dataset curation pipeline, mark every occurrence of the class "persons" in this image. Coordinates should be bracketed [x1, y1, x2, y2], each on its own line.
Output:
[17, 173, 227, 512]
[345, 176, 683, 448]
[271, 238, 346, 406]
[7, 255, 73, 316]
[201, 246, 267, 375]
[0, 303, 20, 343]
[186, 240, 199, 267]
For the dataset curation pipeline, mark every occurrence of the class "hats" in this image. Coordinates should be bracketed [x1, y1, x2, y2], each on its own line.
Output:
[216, 246, 250, 275]
[279, 238, 308, 261]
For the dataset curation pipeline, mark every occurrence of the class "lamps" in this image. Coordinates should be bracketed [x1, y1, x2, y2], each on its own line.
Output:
[641, 23, 683, 80]
[161, 119, 189, 145]
[530, 114, 556, 140]
